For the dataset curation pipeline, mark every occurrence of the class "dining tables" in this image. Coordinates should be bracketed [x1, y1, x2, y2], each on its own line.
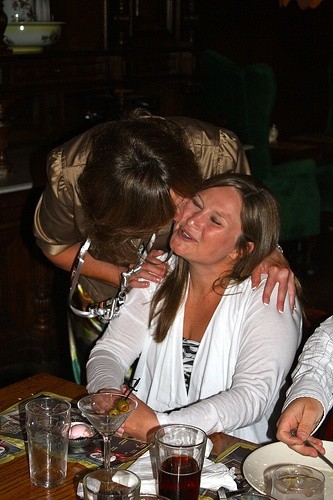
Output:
[0, 371, 275, 500]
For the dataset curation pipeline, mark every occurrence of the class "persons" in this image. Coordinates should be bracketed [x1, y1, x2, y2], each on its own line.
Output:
[86, 175, 302, 445]
[275, 314, 333, 456]
[34, 108, 297, 388]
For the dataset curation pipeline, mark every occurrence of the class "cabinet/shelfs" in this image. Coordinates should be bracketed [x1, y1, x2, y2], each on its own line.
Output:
[0, 0, 200, 372]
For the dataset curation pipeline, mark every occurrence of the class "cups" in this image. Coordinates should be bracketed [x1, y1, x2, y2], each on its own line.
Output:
[271, 464, 325, 500]
[25, 397, 71, 489]
[128, 493, 171, 500]
[83, 467, 141, 500]
[154, 424, 208, 500]
[225, 493, 277, 500]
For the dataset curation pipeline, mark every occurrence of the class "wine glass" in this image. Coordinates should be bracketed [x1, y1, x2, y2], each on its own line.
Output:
[77, 392, 138, 484]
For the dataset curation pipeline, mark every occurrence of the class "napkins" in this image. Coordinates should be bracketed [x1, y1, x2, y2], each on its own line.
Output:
[114, 438, 238, 495]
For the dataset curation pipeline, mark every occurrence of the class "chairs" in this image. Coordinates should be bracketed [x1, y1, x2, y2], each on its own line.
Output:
[201, 50, 322, 238]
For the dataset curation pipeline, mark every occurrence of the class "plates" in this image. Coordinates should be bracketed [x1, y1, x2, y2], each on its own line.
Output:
[243, 439, 333, 500]
